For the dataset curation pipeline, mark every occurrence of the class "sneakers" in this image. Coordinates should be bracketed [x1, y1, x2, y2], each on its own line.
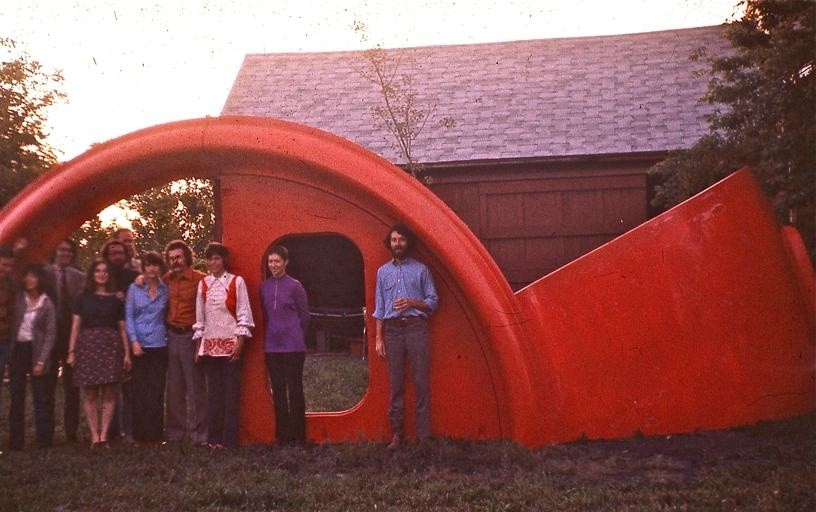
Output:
[388, 434, 403, 451]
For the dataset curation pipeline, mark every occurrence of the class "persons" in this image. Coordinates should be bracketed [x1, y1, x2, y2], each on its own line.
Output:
[259, 245, 311, 444]
[372, 225, 440, 450]
[189, 242, 256, 446]
[88, 241, 142, 298]
[123, 251, 169, 448]
[134, 239, 207, 445]
[113, 228, 141, 276]
[5, 239, 58, 448]
[66, 259, 132, 447]
[0, 245, 16, 382]
[11, 239, 85, 441]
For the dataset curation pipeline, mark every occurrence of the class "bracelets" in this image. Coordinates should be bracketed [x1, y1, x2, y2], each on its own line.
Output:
[67, 348, 73, 354]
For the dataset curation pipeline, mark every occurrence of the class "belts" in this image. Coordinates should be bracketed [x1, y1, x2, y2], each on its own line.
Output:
[386, 317, 422, 324]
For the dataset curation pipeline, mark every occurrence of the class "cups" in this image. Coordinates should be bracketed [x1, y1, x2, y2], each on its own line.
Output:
[392, 296, 401, 311]
